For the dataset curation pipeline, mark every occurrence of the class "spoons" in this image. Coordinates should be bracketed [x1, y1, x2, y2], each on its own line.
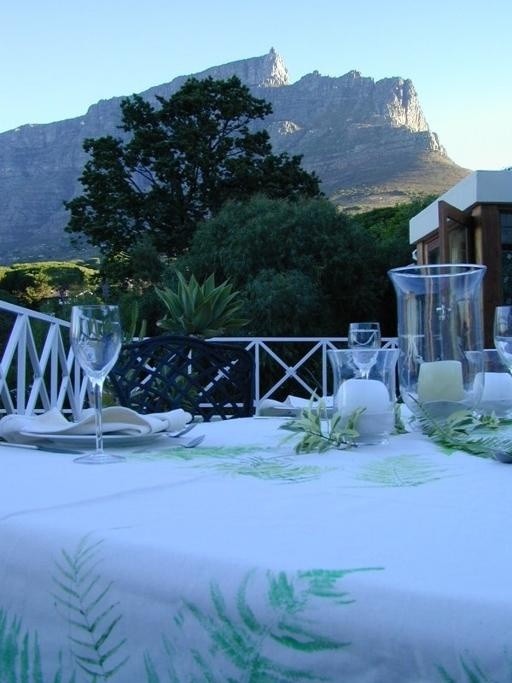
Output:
[172, 434, 209, 448]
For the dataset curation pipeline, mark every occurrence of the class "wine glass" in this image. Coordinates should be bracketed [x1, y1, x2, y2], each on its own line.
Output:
[70, 305, 123, 465]
[343, 324, 385, 379]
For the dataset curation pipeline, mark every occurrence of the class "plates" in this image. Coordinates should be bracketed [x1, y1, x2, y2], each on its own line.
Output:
[11, 426, 164, 448]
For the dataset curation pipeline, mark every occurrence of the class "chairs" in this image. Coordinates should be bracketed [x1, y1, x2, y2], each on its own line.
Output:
[108, 336, 256, 422]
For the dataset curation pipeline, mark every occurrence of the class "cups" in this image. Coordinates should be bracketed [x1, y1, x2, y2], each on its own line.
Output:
[494, 307, 512, 375]
[324, 349, 402, 448]
[387, 265, 485, 418]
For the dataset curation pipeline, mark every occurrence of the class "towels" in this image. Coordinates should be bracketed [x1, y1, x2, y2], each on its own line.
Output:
[0, 406, 192, 445]
